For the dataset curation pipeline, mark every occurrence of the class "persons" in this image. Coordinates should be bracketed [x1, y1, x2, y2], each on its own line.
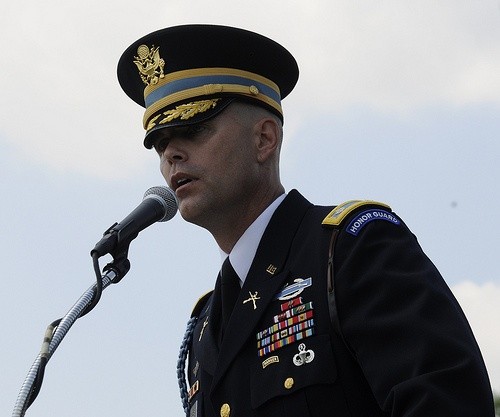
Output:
[117, 24, 498, 417]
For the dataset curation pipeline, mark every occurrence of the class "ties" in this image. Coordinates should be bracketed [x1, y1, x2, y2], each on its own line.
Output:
[221, 257, 241, 340]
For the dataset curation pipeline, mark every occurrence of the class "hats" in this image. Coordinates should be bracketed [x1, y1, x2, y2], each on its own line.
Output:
[117, 24, 299, 150]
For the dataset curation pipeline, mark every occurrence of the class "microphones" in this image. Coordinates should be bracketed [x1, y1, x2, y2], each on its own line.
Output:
[91, 185, 179, 258]
